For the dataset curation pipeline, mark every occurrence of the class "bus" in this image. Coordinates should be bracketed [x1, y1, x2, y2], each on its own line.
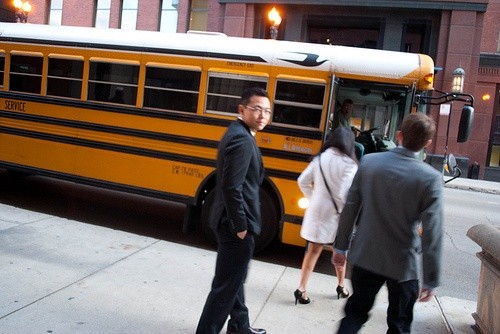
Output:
[0, 21, 475, 257]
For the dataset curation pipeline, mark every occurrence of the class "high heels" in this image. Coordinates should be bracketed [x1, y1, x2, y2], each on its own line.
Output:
[294, 289, 310, 305]
[337, 285, 350, 299]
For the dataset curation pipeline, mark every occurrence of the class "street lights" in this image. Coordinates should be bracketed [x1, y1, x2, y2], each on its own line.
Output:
[267, 6, 283, 40]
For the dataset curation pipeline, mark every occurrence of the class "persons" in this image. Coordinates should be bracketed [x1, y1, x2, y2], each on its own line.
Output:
[336, 99, 364, 161]
[293, 128, 359, 305]
[330, 112, 444, 334]
[196, 87, 272, 334]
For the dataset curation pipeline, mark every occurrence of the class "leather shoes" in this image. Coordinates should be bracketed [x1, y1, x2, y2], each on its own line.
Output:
[245, 327, 266, 334]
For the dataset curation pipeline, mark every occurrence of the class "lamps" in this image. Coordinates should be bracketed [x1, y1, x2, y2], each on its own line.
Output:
[452, 67, 465, 93]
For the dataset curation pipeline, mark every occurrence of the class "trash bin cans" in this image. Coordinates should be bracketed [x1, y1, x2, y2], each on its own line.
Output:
[467, 223, 500, 334]
[468, 163, 480, 178]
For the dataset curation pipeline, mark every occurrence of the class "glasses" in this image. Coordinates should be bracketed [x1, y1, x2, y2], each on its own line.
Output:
[245, 105, 272, 116]
